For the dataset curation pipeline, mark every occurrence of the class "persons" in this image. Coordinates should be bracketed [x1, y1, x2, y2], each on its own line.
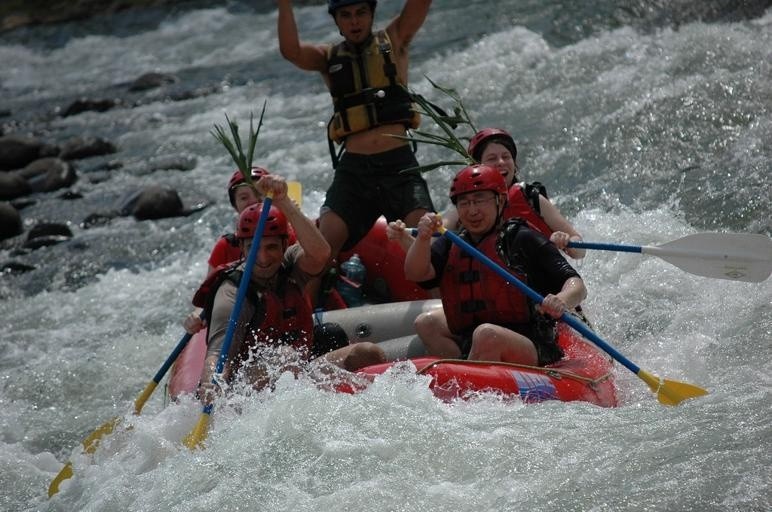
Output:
[197, 174, 387, 408]
[278, 0, 437, 304]
[405, 166, 589, 366]
[468, 127, 588, 262]
[180, 166, 300, 334]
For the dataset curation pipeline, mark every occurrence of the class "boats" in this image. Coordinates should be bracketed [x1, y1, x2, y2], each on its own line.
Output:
[168, 215, 611, 442]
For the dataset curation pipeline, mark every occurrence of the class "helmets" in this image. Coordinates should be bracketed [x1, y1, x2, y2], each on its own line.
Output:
[238, 202, 287, 238]
[228, 167, 269, 205]
[450, 164, 509, 204]
[468, 127, 517, 159]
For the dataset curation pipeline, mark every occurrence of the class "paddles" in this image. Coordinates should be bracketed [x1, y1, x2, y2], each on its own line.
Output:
[47, 314, 207, 498]
[440, 226, 710, 404]
[563, 235, 771, 282]
[182, 188, 275, 447]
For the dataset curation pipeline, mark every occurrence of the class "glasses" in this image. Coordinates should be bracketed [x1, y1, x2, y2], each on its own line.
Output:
[457, 197, 496, 208]
